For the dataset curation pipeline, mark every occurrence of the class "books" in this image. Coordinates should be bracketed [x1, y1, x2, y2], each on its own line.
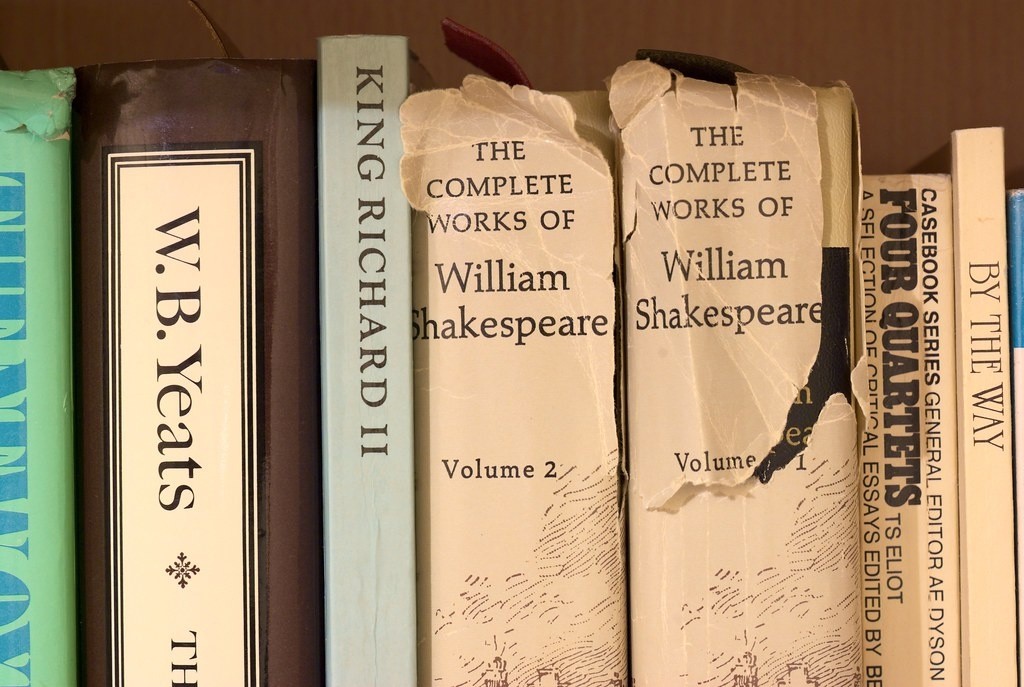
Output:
[0, 34, 1024, 686]
